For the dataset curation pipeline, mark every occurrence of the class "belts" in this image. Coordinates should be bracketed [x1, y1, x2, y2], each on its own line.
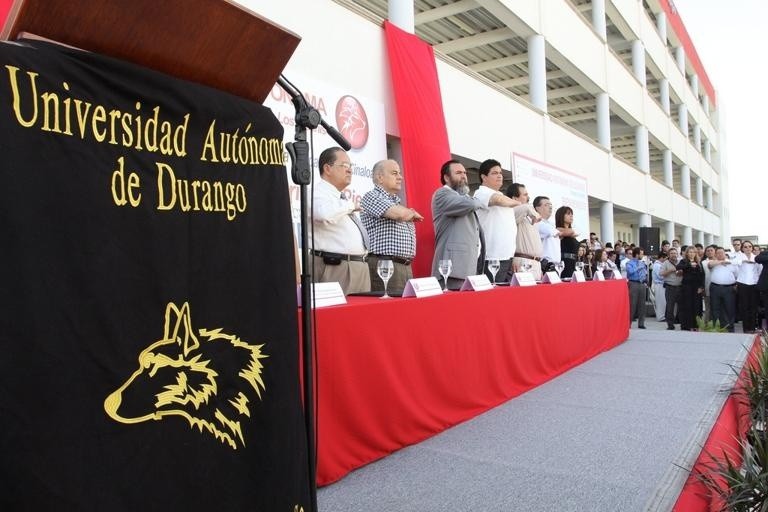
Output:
[309, 250, 367, 261]
[631, 280, 646, 283]
[515, 253, 542, 261]
[711, 282, 736, 286]
[368, 253, 412, 265]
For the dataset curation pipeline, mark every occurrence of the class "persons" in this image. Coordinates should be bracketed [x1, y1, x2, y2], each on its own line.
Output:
[358, 158, 425, 295]
[529, 195, 575, 278]
[306, 145, 372, 294]
[504, 181, 544, 282]
[429, 159, 490, 291]
[554, 204, 581, 281]
[571, 231, 767, 334]
[471, 158, 522, 283]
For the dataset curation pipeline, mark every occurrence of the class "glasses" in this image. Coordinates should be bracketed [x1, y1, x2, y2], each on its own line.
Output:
[733, 244, 740, 246]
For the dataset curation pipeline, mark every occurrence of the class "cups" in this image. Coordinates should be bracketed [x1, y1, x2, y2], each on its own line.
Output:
[520, 258, 533, 272]
[575, 262, 584, 271]
[597, 263, 606, 272]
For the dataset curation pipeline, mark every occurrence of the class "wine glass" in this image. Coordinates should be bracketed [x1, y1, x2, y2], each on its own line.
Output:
[554, 261, 565, 277]
[438, 259, 453, 293]
[377, 260, 395, 298]
[488, 258, 500, 287]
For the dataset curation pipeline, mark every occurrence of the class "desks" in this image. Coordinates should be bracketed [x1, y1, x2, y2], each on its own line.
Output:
[294, 277, 632, 489]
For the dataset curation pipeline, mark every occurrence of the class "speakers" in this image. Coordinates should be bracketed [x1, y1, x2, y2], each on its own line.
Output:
[640, 226, 660, 255]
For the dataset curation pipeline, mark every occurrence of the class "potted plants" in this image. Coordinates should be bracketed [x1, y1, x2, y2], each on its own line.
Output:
[714, 335, 768, 466]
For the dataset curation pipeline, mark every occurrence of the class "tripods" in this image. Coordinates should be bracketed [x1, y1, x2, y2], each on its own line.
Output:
[644, 256, 657, 319]
